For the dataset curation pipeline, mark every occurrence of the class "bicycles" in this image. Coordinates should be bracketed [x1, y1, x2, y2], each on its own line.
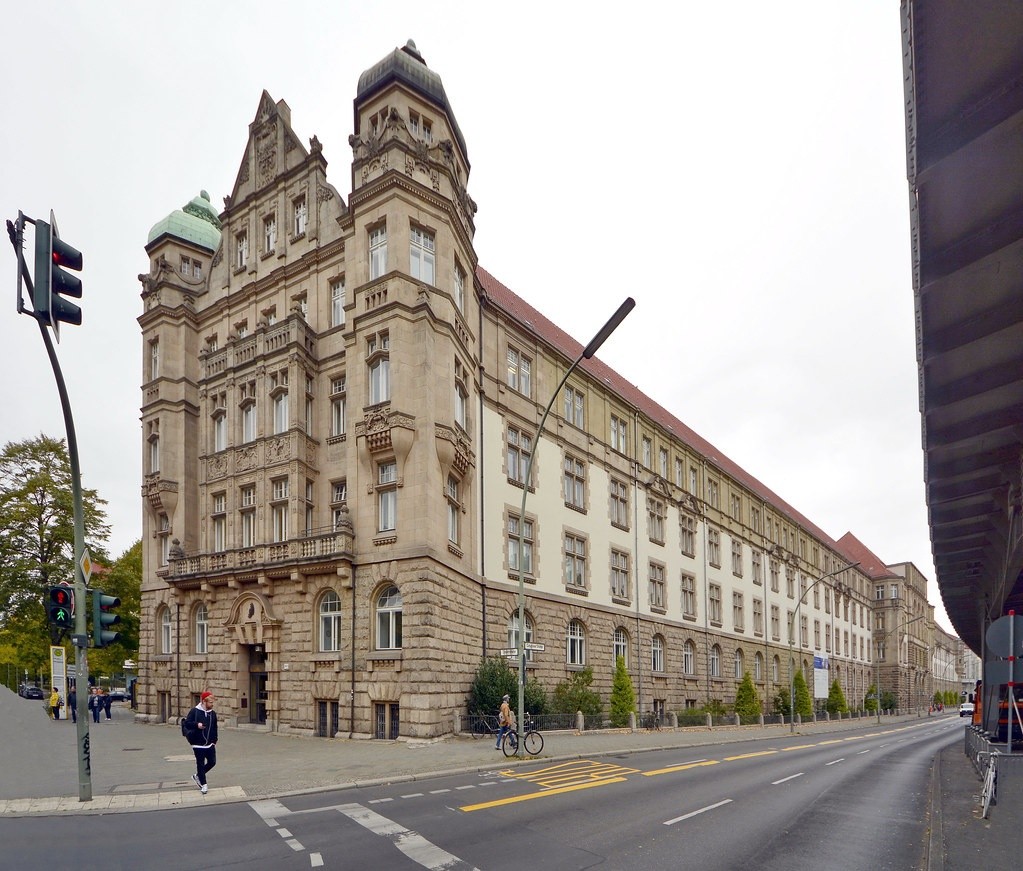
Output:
[470, 709, 501, 740]
[973, 750, 997, 818]
[502, 711, 544, 757]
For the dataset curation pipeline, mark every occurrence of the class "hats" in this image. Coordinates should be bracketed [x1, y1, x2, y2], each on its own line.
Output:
[503, 695, 510, 699]
[202, 692, 212, 700]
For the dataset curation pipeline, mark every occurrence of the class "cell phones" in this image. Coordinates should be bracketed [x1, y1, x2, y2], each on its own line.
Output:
[202, 724, 207, 726]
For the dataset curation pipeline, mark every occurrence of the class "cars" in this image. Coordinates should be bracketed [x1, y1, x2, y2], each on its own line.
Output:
[23, 687, 43, 699]
[959, 703, 975, 717]
[107, 690, 132, 703]
[88, 681, 91, 688]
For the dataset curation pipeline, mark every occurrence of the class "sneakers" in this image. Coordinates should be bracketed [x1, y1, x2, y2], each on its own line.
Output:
[191, 774, 202, 788]
[201, 785, 208, 793]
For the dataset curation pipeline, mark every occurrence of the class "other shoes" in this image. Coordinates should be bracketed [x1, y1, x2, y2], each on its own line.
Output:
[72, 721, 76, 723]
[495, 747, 501, 750]
[105, 718, 111, 721]
[94, 720, 99, 723]
[54, 718, 58, 720]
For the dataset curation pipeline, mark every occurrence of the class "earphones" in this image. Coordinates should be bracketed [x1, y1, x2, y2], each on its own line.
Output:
[204, 702, 206, 704]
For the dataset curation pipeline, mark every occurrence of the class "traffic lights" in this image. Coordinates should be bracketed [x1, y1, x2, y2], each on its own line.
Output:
[92, 588, 121, 648]
[50, 587, 72, 628]
[35, 209, 83, 343]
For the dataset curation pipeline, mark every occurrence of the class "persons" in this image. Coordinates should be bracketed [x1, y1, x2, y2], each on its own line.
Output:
[67, 686, 76, 723]
[186, 691, 218, 793]
[88, 688, 112, 723]
[50, 687, 61, 720]
[496, 695, 518, 750]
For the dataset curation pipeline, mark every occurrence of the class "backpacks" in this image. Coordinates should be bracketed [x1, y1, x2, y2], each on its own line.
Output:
[57, 695, 64, 706]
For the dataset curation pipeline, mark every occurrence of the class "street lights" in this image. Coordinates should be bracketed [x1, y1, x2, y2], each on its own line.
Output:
[877, 615, 925, 724]
[942, 653, 972, 713]
[928, 638, 962, 717]
[790, 561, 861, 734]
[517, 296, 638, 756]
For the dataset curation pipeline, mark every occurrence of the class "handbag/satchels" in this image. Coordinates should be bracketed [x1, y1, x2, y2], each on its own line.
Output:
[181, 708, 197, 737]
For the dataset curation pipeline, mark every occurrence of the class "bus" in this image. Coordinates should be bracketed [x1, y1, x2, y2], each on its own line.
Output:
[99, 677, 126, 692]
